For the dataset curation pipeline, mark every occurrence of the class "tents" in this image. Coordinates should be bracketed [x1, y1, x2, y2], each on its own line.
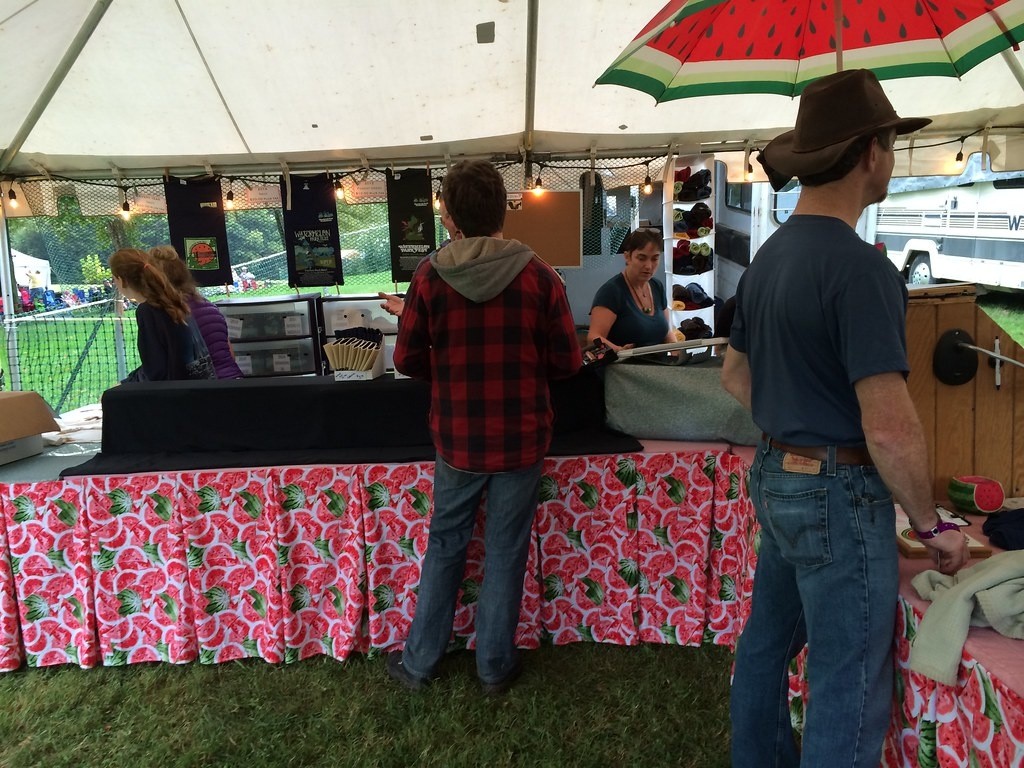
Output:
[11, 249, 52, 291]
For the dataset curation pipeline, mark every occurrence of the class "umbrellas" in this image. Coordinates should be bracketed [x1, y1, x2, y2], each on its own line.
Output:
[591, 0, 1023, 108]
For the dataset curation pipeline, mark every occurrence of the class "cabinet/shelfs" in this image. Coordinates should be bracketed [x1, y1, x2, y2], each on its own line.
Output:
[214, 292, 406, 378]
[663, 153, 716, 356]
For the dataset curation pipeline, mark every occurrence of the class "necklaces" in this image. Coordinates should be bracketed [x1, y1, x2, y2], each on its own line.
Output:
[625, 270, 653, 313]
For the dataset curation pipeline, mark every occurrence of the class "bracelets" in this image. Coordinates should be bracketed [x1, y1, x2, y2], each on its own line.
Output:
[909, 512, 960, 538]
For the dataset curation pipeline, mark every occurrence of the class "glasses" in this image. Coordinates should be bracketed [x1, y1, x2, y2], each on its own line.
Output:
[631, 228, 663, 237]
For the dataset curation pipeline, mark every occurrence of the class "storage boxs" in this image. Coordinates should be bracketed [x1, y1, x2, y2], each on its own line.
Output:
[0, 391, 62, 465]
[334, 334, 386, 381]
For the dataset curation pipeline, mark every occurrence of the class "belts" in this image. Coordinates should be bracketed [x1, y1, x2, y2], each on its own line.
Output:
[761, 430, 874, 464]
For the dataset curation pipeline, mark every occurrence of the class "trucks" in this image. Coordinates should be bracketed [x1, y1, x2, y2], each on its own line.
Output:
[854, 160, 1024, 290]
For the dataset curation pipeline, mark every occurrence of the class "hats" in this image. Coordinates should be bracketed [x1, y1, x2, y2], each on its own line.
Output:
[757, 69, 933, 191]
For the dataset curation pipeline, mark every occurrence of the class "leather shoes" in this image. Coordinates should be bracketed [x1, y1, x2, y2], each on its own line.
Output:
[387, 655, 426, 690]
[481, 656, 525, 694]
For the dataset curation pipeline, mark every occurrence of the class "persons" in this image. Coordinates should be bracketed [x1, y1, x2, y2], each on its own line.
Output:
[587, 229, 680, 359]
[721, 68, 971, 768]
[0, 246, 255, 384]
[379, 158, 581, 696]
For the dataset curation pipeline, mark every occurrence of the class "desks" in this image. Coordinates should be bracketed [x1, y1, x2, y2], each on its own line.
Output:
[0, 403, 1024, 768]
